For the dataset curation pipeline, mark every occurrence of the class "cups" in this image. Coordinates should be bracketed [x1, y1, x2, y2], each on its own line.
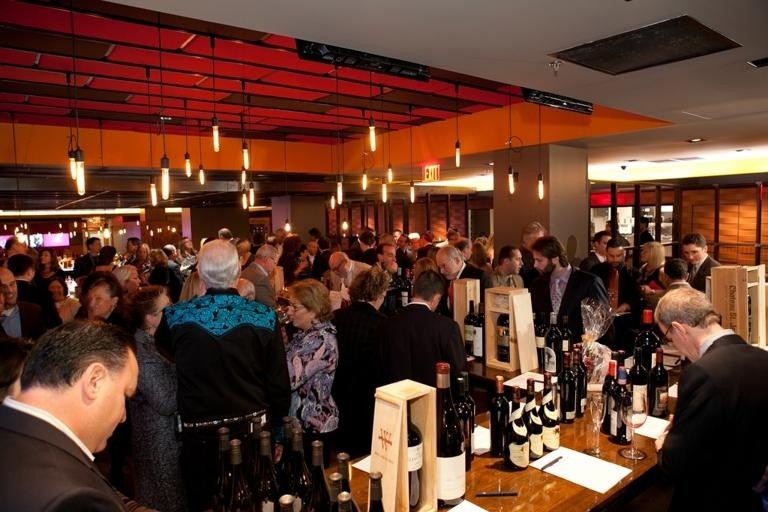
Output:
[621, 391, 648, 460]
[278, 312, 291, 328]
[584, 393, 606, 456]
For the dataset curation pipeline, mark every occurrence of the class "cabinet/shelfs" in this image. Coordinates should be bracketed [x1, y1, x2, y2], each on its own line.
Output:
[467, 360, 567, 415]
[323, 349, 734, 512]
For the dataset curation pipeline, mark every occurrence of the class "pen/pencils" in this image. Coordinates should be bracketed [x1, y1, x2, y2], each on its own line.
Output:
[475, 492, 518, 497]
[541, 457, 562, 470]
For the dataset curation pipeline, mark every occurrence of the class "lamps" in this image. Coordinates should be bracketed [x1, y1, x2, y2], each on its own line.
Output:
[67, 71, 78, 181]
[241, 182, 247, 210]
[507, 99, 514, 195]
[362, 109, 368, 191]
[249, 133, 255, 207]
[284, 137, 291, 233]
[41, 218, 91, 248]
[210, 37, 220, 153]
[409, 106, 415, 204]
[70, 10, 85, 196]
[336, 67, 343, 205]
[342, 218, 349, 231]
[12, 114, 26, 244]
[157, 18, 169, 201]
[387, 123, 393, 184]
[198, 120, 205, 185]
[538, 106, 544, 201]
[330, 132, 335, 210]
[369, 71, 376, 152]
[117, 215, 178, 242]
[240, 80, 249, 169]
[183, 99, 192, 178]
[381, 85, 387, 204]
[240, 130, 246, 185]
[455, 83, 461, 168]
[99, 118, 110, 240]
[145, 66, 157, 207]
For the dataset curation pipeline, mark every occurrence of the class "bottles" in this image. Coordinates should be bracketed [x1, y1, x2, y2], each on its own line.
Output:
[504, 386, 530, 470]
[610, 366, 632, 444]
[464, 300, 477, 354]
[536, 312, 546, 365]
[213, 428, 231, 512]
[497, 313, 510, 362]
[454, 378, 472, 471]
[247, 431, 279, 512]
[560, 315, 572, 353]
[337, 452, 360, 512]
[393, 267, 404, 287]
[286, 428, 311, 512]
[306, 441, 330, 512]
[405, 269, 413, 298]
[576, 343, 588, 386]
[406, 422, 423, 472]
[460, 371, 475, 461]
[572, 349, 586, 418]
[472, 303, 485, 362]
[221, 439, 250, 512]
[435, 362, 466, 509]
[630, 348, 648, 414]
[522, 378, 544, 461]
[338, 491, 352, 512]
[272, 417, 292, 480]
[552, 376, 560, 418]
[649, 348, 669, 418]
[616, 350, 631, 392]
[279, 494, 294, 512]
[634, 309, 661, 371]
[490, 375, 510, 458]
[538, 372, 560, 451]
[247, 417, 262, 442]
[543, 312, 562, 376]
[558, 352, 577, 424]
[600, 359, 616, 435]
[369, 472, 384, 512]
[329, 473, 342, 512]
[399, 285, 409, 311]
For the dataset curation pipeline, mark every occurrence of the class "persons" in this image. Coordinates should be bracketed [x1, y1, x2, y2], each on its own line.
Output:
[393, 229, 402, 244]
[134, 244, 152, 275]
[608, 220, 631, 258]
[276, 229, 287, 242]
[153, 239, 291, 466]
[351, 227, 374, 248]
[90, 271, 133, 333]
[519, 220, 546, 287]
[453, 237, 472, 260]
[590, 238, 638, 317]
[323, 271, 351, 311]
[390, 270, 465, 388]
[526, 235, 615, 346]
[179, 236, 215, 301]
[265, 233, 283, 258]
[436, 245, 493, 317]
[327, 267, 411, 458]
[329, 252, 372, 291]
[237, 278, 255, 301]
[663, 258, 692, 291]
[379, 233, 396, 245]
[235, 240, 256, 270]
[0, 330, 33, 402]
[74, 237, 101, 279]
[128, 286, 178, 511]
[241, 244, 278, 307]
[177, 238, 198, 277]
[162, 244, 189, 282]
[414, 257, 438, 279]
[312, 237, 331, 281]
[250, 233, 265, 253]
[75, 275, 89, 296]
[8, 253, 62, 325]
[489, 245, 524, 290]
[578, 231, 611, 270]
[635, 217, 654, 245]
[90, 245, 118, 274]
[638, 241, 666, 296]
[0, 269, 45, 340]
[0, 318, 160, 512]
[279, 235, 301, 265]
[417, 231, 434, 258]
[350, 231, 377, 265]
[284, 279, 340, 470]
[218, 228, 238, 244]
[293, 240, 318, 281]
[139, 249, 169, 286]
[125, 237, 140, 266]
[50, 278, 81, 322]
[397, 234, 411, 256]
[446, 225, 460, 244]
[376, 242, 398, 273]
[681, 233, 722, 294]
[654, 288, 768, 512]
[467, 243, 492, 274]
[37, 249, 64, 287]
[112, 265, 140, 316]
[5, 236, 29, 255]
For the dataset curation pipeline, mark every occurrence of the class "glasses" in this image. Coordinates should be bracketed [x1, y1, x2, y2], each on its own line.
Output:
[662, 325, 672, 344]
